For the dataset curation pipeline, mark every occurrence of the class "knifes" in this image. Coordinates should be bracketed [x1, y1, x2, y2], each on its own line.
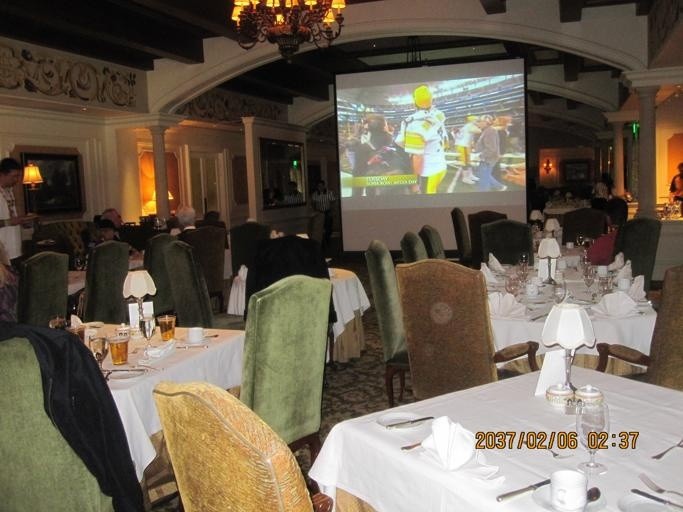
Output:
[496, 478, 551, 502]
[386, 416, 434, 428]
[630, 488, 683, 509]
[109, 368, 147, 372]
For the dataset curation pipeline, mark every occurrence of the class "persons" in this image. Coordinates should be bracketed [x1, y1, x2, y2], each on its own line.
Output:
[339, 83, 525, 196]
[100, 208, 122, 227]
[310, 180, 335, 244]
[582, 198, 628, 265]
[285, 181, 303, 205]
[170, 206, 197, 235]
[0, 241, 20, 293]
[668, 162, 683, 204]
[0, 158, 43, 271]
[94, 219, 140, 263]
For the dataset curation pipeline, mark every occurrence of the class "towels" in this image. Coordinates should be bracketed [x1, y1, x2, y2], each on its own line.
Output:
[143, 337, 178, 359]
[417, 416, 480, 473]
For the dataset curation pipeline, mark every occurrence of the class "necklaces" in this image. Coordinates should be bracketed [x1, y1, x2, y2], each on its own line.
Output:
[0, 188, 16, 218]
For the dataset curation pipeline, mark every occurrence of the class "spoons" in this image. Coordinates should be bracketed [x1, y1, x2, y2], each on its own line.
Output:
[582, 487, 601, 511]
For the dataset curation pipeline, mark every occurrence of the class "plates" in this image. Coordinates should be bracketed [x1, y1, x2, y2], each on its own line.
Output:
[105, 366, 144, 380]
[376, 412, 423, 431]
[532, 482, 607, 512]
[617, 491, 683, 512]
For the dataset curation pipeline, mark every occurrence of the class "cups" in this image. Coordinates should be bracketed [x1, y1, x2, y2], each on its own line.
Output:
[618, 279, 630, 291]
[187, 328, 204, 343]
[109, 337, 130, 365]
[575, 384, 603, 406]
[531, 277, 543, 287]
[526, 284, 538, 296]
[535, 470, 588, 511]
[116, 323, 131, 337]
[545, 383, 574, 407]
[157, 314, 177, 341]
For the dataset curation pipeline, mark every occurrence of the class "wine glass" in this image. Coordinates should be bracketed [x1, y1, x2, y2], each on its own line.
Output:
[553, 281, 566, 303]
[139, 314, 156, 347]
[75, 256, 84, 280]
[576, 233, 584, 247]
[598, 277, 612, 297]
[516, 263, 529, 283]
[579, 237, 594, 262]
[89, 333, 109, 369]
[574, 401, 610, 476]
[504, 277, 517, 293]
[518, 252, 530, 265]
[584, 263, 595, 292]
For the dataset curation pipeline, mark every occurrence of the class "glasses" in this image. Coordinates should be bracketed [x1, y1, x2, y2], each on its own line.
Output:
[359, 128, 369, 134]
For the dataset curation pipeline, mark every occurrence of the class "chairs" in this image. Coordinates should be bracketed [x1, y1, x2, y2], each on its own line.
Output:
[152, 380, 332, 512]
[258, 234, 337, 365]
[0, 321, 146, 512]
[230, 273, 334, 496]
[0, 214, 270, 330]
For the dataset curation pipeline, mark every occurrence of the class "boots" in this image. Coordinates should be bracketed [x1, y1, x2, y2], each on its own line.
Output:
[462, 170, 475, 184]
[468, 167, 480, 181]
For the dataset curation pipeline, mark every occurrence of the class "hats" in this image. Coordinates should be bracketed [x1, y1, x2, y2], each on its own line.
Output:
[414, 85, 433, 108]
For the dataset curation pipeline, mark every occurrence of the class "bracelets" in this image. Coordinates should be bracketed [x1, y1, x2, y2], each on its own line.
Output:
[3, 219, 10, 226]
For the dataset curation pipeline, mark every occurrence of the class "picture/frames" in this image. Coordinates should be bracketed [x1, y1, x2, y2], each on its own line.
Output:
[19, 150, 84, 217]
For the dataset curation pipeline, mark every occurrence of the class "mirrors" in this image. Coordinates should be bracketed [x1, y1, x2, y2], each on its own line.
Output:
[259, 136, 308, 210]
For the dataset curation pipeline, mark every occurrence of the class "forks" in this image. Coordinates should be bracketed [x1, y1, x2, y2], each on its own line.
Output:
[637, 471, 683, 498]
[652, 439, 683, 460]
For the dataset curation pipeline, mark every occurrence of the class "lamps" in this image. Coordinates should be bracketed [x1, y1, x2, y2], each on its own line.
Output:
[152, 190, 174, 200]
[231, 0, 348, 66]
[22, 164, 44, 191]
[544, 159, 552, 176]
